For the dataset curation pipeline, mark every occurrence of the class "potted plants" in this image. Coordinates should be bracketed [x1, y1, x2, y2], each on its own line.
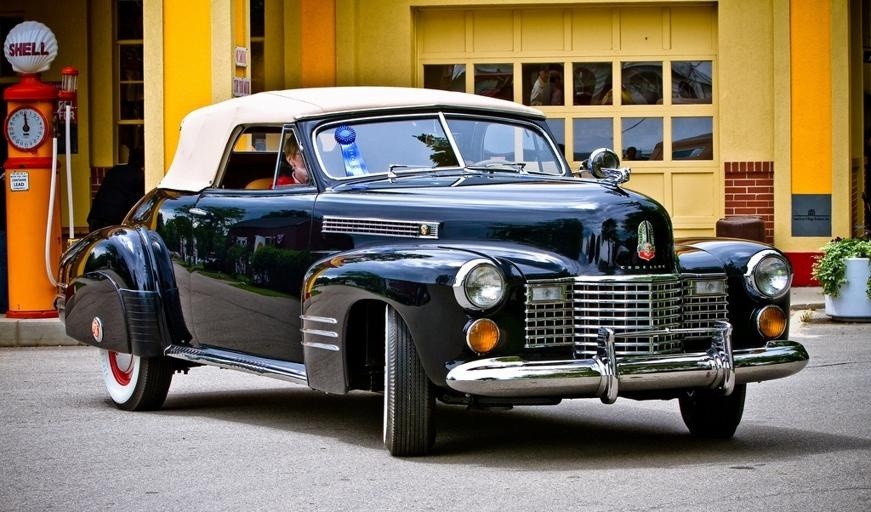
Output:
[811, 236, 871, 323]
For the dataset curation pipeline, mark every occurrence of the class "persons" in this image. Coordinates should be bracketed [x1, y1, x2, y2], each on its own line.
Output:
[268, 133, 324, 190]
[530, 64, 551, 106]
[87, 147, 145, 233]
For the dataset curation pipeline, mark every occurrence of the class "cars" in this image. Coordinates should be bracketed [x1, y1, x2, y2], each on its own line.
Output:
[54, 86, 810, 459]
